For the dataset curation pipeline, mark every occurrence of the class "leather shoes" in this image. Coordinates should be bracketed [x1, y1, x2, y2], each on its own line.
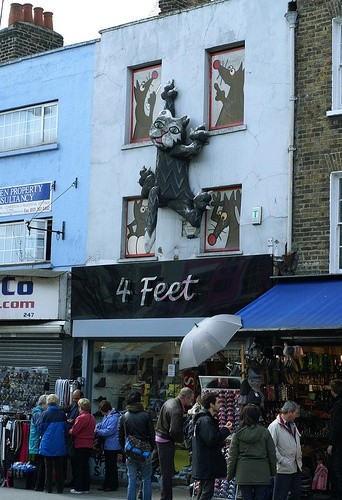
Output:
[57, 488, 63, 493]
[44, 488, 53, 493]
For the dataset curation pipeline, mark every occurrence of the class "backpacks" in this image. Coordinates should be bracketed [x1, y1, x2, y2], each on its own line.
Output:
[280, 353, 339, 376]
[311, 463, 328, 490]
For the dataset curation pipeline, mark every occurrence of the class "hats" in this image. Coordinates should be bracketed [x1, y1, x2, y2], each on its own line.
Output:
[237, 396, 248, 405]
[256, 351, 265, 365]
[248, 363, 269, 381]
[187, 402, 201, 415]
[249, 347, 258, 361]
[247, 378, 263, 392]
[293, 346, 306, 357]
[263, 346, 275, 360]
[72, 390, 84, 399]
[46, 394, 60, 404]
[271, 345, 286, 357]
[239, 379, 252, 395]
[126, 391, 142, 405]
[283, 345, 295, 357]
[38, 394, 47, 403]
[247, 391, 265, 403]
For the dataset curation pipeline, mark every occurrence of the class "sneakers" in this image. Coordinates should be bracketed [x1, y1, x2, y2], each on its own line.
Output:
[93, 396, 106, 403]
[83, 490, 90, 494]
[69, 488, 82, 494]
[93, 410, 104, 417]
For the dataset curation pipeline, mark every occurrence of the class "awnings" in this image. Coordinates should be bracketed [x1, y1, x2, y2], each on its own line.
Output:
[230, 281, 341, 333]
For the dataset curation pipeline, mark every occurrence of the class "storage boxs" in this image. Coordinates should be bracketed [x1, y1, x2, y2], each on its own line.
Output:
[14, 473, 32, 489]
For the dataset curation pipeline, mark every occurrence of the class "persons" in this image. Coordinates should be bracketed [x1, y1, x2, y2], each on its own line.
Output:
[28, 387, 306, 500]
[325, 378, 342, 500]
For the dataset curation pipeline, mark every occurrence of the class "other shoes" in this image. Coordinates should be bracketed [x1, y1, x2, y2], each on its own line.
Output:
[35, 486, 45, 491]
[133, 381, 143, 387]
[104, 487, 117, 492]
[97, 486, 104, 491]
[137, 491, 142, 499]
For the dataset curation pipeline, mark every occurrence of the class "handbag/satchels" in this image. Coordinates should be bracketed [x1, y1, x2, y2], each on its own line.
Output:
[173, 445, 191, 473]
[94, 432, 105, 453]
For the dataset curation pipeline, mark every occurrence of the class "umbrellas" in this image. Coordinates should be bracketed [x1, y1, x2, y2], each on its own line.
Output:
[177, 313, 242, 371]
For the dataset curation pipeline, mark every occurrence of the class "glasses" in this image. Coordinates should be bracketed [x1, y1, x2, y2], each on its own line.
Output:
[214, 391, 241, 427]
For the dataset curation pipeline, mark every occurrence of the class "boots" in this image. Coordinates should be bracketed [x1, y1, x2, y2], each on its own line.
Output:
[154, 359, 164, 369]
[118, 353, 130, 374]
[146, 357, 154, 373]
[95, 377, 106, 387]
[116, 396, 125, 411]
[128, 354, 140, 375]
[107, 352, 120, 373]
[94, 351, 107, 373]
[138, 357, 145, 370]
